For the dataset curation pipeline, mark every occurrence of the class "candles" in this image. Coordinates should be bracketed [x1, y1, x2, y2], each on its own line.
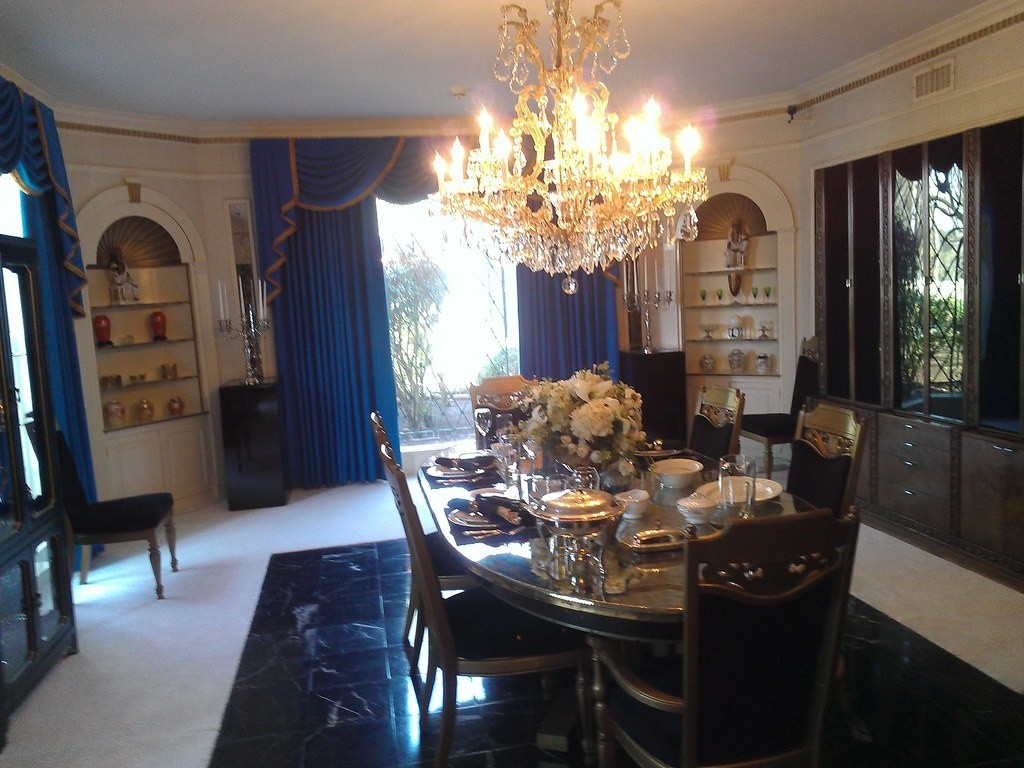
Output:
[216, 273, 270, 324]
[619, 254, 675, 300]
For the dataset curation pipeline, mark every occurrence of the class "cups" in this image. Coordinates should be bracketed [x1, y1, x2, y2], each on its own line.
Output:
[716, 453, 757, 522]
[545, 473, 566, 495]
[572, 466, 600, 489]
[614, 489, 650, 519]
[161, 362, 176, 379]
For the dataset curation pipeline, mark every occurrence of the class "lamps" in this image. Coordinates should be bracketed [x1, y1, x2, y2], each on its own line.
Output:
[424, 0, 708, 294]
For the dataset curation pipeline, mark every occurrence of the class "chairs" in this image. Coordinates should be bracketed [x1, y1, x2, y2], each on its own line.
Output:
[587, 500, 859, 766]
[369, 408, 590, 766]
[685, 384, 745, 463]
[468, 371, 543, 472]
[25, 419, 176, 597]
[783, 393, 869, 518]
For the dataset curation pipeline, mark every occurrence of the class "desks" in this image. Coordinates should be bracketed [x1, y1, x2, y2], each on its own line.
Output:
[217, 376, 288, 511]
[621, 349, 685, 442]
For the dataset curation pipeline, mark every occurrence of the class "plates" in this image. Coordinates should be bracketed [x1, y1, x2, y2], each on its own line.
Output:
[633, 449, 682, 456]
[696, 476, 783, 506]
[448, 488, 506, 528]
[425, 465, 484, 478]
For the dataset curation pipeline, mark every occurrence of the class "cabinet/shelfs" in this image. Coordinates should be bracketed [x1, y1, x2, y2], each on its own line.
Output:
[675, 235, 780, 376]
[1, 234, 79, 749]
[81, 260, 208, 434]
[807, 114, 1023, 594]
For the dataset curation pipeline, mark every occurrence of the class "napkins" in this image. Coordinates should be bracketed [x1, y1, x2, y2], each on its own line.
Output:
[434, 454, 499, 476]
[447, 492, 537, 529]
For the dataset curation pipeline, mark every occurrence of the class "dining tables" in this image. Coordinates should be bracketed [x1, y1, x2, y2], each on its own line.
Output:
[416, 438, 834, 767]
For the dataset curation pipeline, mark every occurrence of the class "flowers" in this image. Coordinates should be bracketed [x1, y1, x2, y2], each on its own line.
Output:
[494, 359, 643, 475]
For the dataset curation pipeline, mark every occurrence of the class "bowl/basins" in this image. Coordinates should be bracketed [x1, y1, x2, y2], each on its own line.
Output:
[676, 492, 718, 525]
[526, 488, 627, 536]
[649, 458, 704, 489]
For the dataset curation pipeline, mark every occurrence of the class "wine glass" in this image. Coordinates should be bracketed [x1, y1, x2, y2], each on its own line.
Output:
[700, 289, 706, 305]
[475, 408, 538, 497]
[728, 273, 741, 305]
[717, 289, 723, 305]
[765, 287, 771, 304]
[752, 287, 758, 304]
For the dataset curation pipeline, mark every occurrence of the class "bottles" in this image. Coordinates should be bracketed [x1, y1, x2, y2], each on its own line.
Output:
[168, 397, 185, 416]
[151, 311, 167, 336]
[104, 400, 126, 428]
[701, 354, 715, 372]
[137, 399, 153, 423]
[755, 353, 770, 373]
[93, 314, 110, 342]
[728, 349, 745, 374]
